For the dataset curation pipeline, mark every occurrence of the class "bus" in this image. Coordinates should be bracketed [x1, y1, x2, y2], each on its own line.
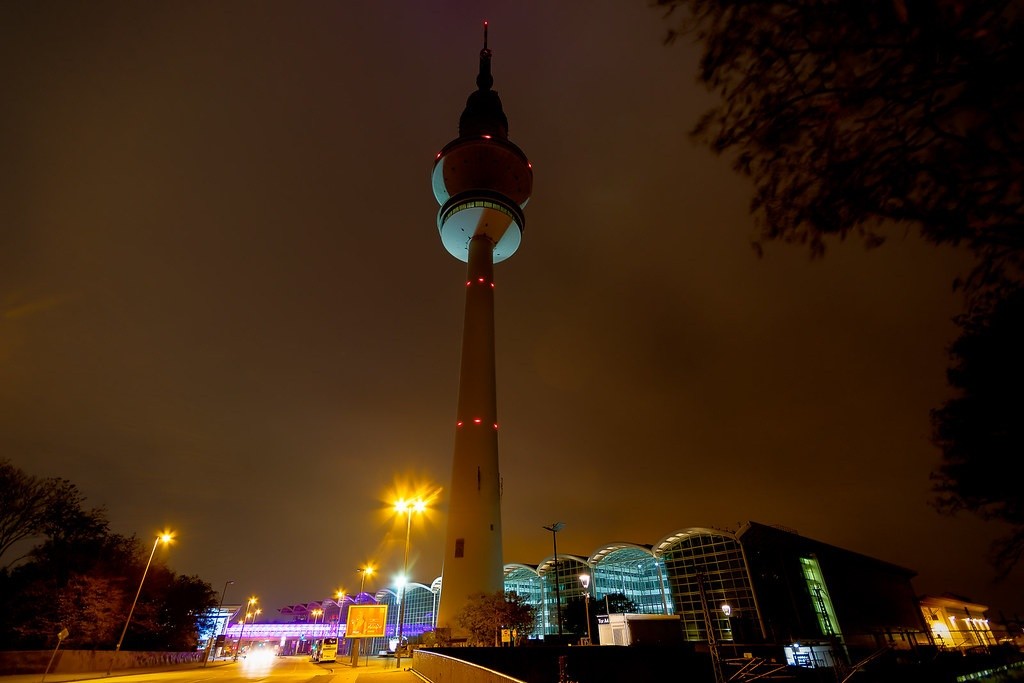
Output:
[312, 638, 339, 664]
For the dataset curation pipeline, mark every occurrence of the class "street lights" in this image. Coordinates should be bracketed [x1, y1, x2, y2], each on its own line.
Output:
[351, 567, 373, 667]
[393, 500, 426, 669]
[578, 573, 593, 646]
[721, 604, 738, 659]
[106, 535, 172, 674]
[234, 598, 256, 663]
[244, 609, 260, 659]
[202, 580, 235, 667]
